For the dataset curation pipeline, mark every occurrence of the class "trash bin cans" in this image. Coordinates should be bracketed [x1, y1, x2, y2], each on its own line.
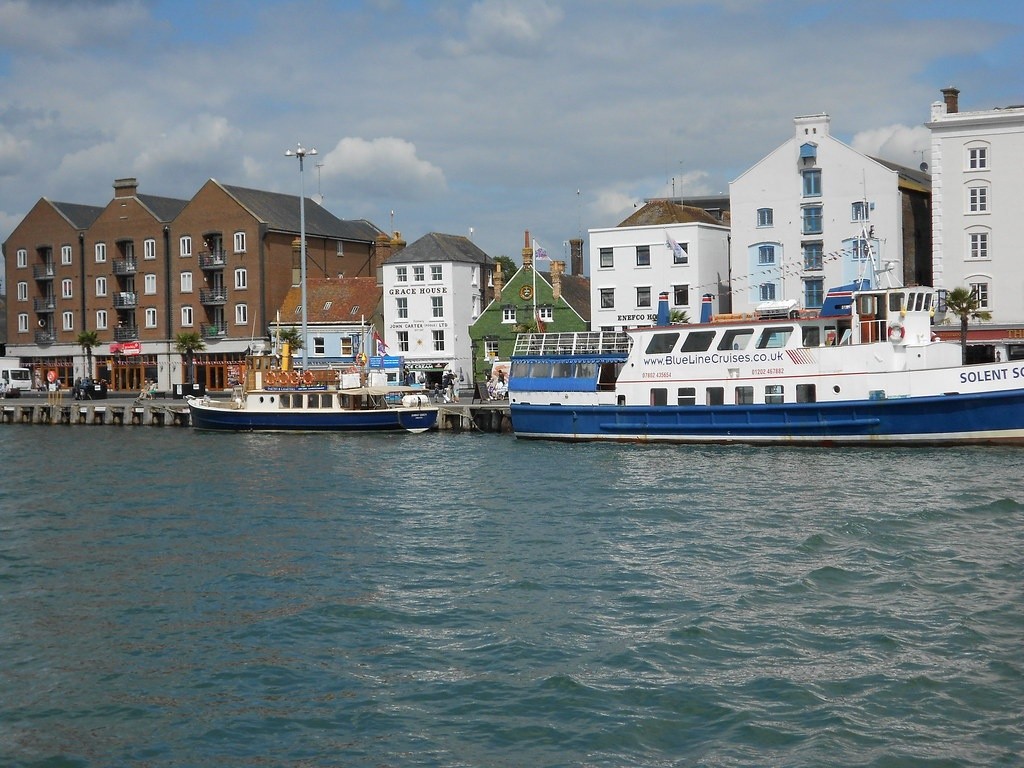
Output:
[81, 384, 106, 399]
[173, 382, 206, 400]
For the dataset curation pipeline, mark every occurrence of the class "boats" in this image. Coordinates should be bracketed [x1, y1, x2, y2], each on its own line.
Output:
[183, 309, 439, 432]
[508, 166, 1024, 444]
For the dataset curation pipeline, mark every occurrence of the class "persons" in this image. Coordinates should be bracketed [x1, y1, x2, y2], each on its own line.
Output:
[36, 375, 42, 391]
[996, 352, 1001, 362]
[138, 381, 155, 400]
[434, 369, 459, 404]
[75, 377, 92, 400]
[487, 372, 492, 382]
[825, 333, 835, 346]
[498, 370, 505, 385]
[0, 383, 11, 399]
[405, 368, 414, 386]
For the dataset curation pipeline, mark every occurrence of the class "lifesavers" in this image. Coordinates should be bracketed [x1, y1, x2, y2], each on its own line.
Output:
[288, 371, 301, 386]
[277, 370, 290, 385]
[302, 370, 315, 385]
[355, 352, 368, 366]
[265, 371, 276, 386]
[888, 321, 905, 343]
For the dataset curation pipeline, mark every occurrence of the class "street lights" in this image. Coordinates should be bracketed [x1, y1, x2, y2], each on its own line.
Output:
[284, 142, 318, 376]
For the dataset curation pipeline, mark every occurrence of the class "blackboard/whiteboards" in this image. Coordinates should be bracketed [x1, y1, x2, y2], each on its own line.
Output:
[473, 381, 490, 400]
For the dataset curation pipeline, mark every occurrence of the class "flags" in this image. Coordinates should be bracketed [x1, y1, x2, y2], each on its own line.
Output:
[666, 230, 689, 258]
[372, 326, 390, 349]
[535, 240, 552, 261]
